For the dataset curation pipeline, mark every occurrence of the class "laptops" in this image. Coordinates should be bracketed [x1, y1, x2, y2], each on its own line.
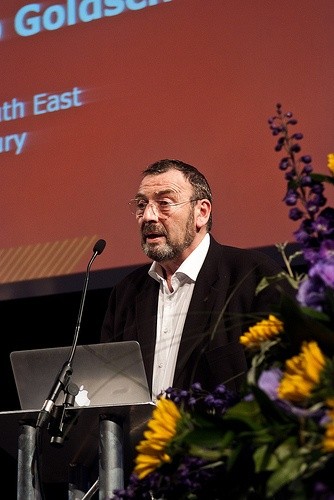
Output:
[10, 342, 152, 410]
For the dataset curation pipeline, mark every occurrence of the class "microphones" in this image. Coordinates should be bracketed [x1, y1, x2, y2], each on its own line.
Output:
[36, 239, 106, 429]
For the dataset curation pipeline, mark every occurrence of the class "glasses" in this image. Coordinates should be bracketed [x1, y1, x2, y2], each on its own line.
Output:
[128, 198, 205, 219]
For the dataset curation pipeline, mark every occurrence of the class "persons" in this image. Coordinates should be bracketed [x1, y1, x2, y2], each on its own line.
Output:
[98, 159, 300, 403]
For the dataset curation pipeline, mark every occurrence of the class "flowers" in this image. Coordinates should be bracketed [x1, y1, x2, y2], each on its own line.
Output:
[111, 103, 334, 500]
